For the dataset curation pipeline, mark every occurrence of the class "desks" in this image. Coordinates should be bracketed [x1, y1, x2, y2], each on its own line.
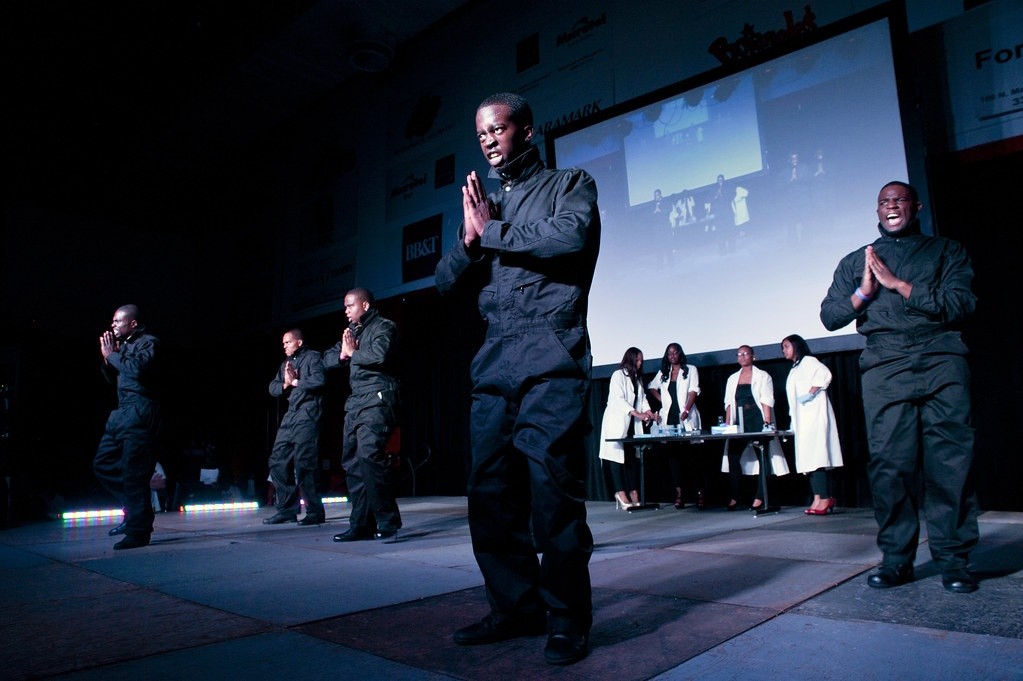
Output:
[605, 430, 796, 516]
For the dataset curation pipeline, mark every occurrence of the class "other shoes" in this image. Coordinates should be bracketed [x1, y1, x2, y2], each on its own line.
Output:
[725, 501, 737, 511]
[748, 501, 764, 512]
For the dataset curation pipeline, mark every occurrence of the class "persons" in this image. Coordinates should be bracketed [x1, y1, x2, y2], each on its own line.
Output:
[648, 342, 706, 511]
[721, 345, 790, 511]
[93, 304, 172, 550]
[263, 328, 325, 526]
[598, 347, 662, 510]
[322, 287, 402, 542]
[652, 174, 750, 253]
[819, 182, 991, 593]
[434, 92, 603, 665]
[781, 334, 844, 515]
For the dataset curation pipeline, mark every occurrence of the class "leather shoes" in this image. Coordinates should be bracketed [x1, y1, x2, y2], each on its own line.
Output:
[262, 513, 297, 524]
[867, 562, 915, 588]
[298, 515, 325, 525]
[545, 625, 590, 665]
[453, 611, 549, 644]
[376, 521, 402, 538]
[108, 520, 128, 534]
[941, 567, 975, 592]
[334, 526, 375, 542]
[113, 533, 150, 550]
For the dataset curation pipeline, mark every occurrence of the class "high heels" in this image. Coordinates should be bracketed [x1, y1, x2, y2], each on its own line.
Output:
[674, 492, 686, 509]
[804, 508, 813, 514]
[810, 497, 835, 516]
[632, 502, 640, 507]
[697, 493, 706, 509]
[614, 493, 634, 510]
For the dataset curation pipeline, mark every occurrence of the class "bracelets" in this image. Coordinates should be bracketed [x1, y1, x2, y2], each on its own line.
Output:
[856, 288, 870, 300]
[686, 411, 689, 413]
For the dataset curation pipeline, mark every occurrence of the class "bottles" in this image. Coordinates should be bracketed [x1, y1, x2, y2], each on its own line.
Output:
[718, 415, 725, 427]
[651, 420, 659, 437]
